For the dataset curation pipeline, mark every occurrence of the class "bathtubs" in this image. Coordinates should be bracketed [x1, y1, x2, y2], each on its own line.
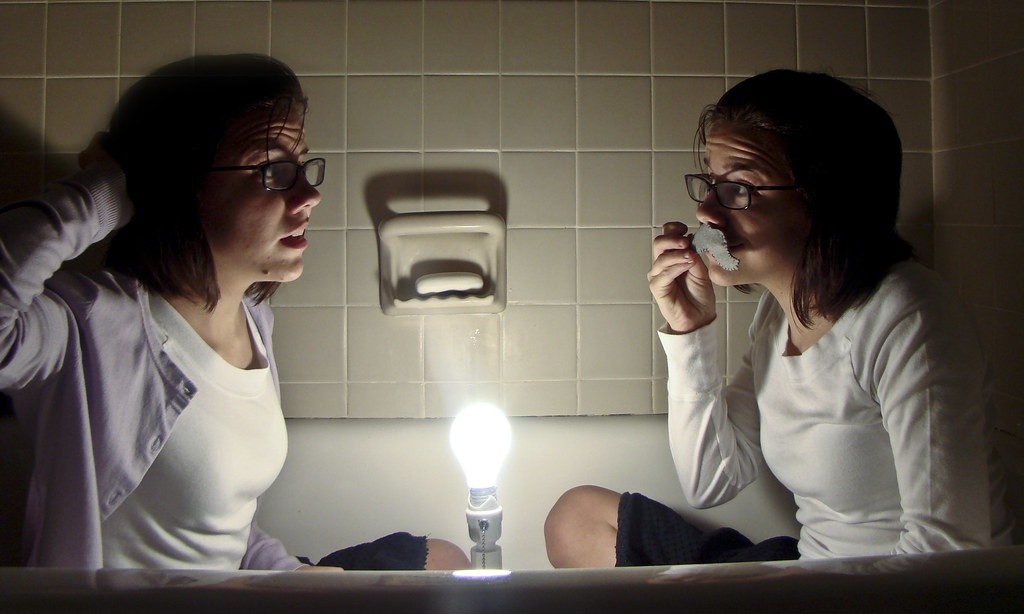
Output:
[0, 415, 1024, 614]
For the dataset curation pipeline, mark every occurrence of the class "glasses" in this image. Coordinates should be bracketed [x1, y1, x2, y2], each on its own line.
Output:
[206, 158, 326, 193]
[685, 172, 794, 210]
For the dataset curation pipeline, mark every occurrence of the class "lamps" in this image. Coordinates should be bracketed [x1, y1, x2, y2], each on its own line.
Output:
[444, 391, 518, 572]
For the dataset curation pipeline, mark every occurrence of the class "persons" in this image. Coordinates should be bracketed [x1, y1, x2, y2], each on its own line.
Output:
[1, 54, 471, 572]
[543, 71, 995, 570]
